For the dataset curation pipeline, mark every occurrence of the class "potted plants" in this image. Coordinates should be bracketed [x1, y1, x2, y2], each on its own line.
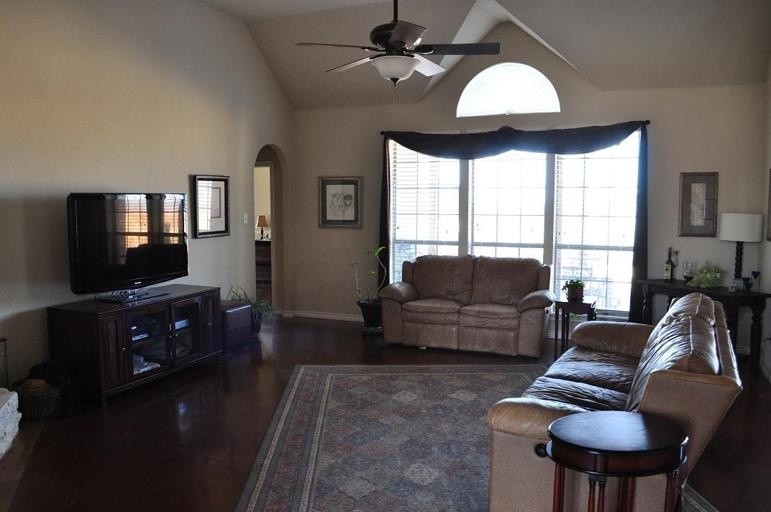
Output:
[232, 285, 278, 339]
[348, 246, 390, 336]
[560, 279, 585, 299]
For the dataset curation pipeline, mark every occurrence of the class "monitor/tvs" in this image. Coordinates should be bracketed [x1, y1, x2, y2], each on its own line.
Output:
[68, 190, 189, 303]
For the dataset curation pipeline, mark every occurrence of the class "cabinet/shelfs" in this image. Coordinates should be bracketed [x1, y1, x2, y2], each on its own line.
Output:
[255, 239, 273, 290]
[47, 283, 223, 410]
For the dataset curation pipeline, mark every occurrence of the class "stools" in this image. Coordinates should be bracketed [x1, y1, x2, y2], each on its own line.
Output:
[221, 300, 253, 351]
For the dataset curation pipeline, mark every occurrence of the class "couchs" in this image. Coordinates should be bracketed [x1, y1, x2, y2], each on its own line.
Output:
[378, 253, 553, 359]
[484, 289, 744, 510]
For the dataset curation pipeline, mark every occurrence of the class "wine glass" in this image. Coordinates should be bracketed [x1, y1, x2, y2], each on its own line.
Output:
[682, 262, 695, 283]
[742, 277, 751, 294]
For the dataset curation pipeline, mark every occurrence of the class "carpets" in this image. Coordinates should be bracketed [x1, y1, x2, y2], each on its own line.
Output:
[234, 364, 717, 511]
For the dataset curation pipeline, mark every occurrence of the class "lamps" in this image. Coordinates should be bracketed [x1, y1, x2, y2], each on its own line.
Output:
[256, 215, 269, 240]
[718, 212, 764, 287]
[369, 55, 420, 105]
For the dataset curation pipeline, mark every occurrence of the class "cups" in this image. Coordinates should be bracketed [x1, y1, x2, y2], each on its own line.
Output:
[728, 280, 736, 292]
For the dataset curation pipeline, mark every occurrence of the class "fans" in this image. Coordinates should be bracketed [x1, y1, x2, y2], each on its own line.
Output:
[291, 1, 501, 79]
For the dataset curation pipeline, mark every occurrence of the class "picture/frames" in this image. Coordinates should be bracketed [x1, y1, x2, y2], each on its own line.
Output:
[678, 171, 719, 238]
[317, 176, 364, 229]
[193, 174, 231, 239]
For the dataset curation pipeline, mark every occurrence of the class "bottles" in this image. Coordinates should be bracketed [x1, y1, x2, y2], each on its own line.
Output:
[663, 247, 674, 283]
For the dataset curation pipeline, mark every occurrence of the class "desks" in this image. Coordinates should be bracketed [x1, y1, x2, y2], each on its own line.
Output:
[553, 296, 599, 359]
[637, 277, 771, 376]
[545, 407, 692, 512]
